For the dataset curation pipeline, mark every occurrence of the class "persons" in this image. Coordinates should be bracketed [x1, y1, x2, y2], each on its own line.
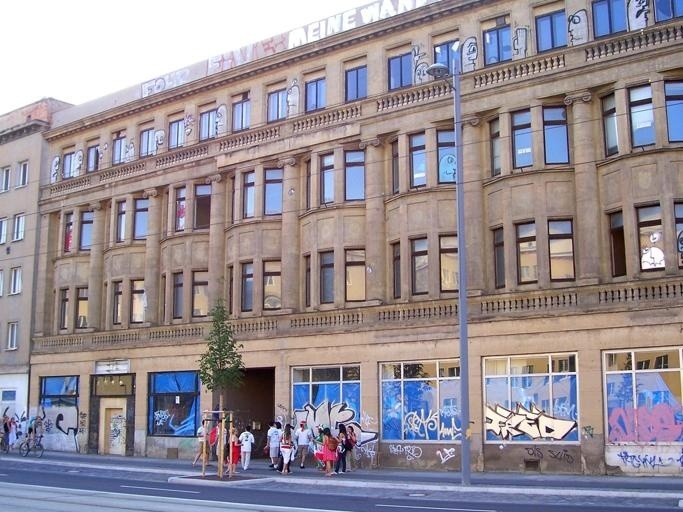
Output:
[191, 418, 357, 477]
[6, 416, 17, 446]
[0, 414, 8, 436]
[3, 415, 8, 445]
[24, 415, 41, 442]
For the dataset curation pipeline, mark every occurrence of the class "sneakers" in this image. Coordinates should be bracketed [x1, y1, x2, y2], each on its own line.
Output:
[300, 464, 304, 469]
[270, 462, 293, 475]
[330, 468, 352, 475]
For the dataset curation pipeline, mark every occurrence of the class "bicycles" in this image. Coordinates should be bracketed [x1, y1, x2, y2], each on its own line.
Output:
[18, 433, 43, 457]
[0, 432, 11, 456]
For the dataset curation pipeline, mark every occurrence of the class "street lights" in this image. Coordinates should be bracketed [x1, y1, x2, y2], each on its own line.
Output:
[426, 45, 472, 486]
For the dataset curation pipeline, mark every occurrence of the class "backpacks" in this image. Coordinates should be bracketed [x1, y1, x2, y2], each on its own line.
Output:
[325, 436, 338, 451]
[337, 432, 353, 452]
